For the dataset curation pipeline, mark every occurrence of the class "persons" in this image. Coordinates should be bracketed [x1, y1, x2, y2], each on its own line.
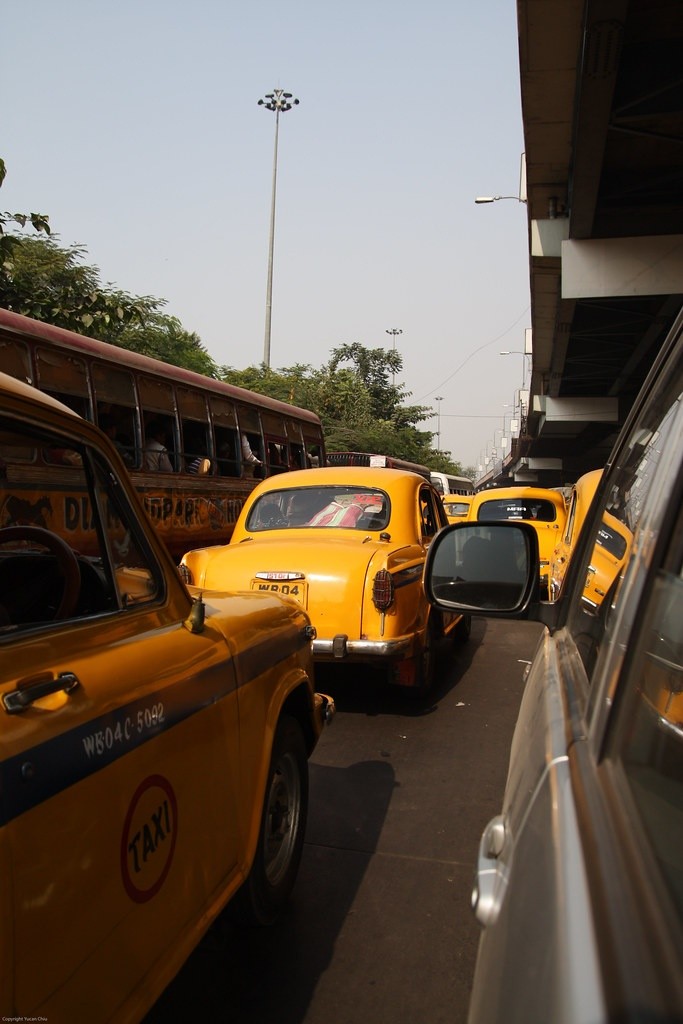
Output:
[98, 413, 136, 468]
[185, 456, 211, 475]
[143, 429, 175, 473]
[260, 503, 285, 528]
[240, 432, 263, 478]
[449, 538, 519, 583]
[222, 440, 232, 458]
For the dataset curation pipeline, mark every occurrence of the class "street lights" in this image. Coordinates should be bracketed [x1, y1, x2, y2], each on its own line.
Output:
[499, 352, 531, 377]
[259, 84, 299, 372]
[434, 396, 444, 450]
[385, 327, 402, 387]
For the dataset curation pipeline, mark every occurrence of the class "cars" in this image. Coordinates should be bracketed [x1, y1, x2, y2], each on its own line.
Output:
[0, 373, 339, 1024]
[421, 493, 475, 536]
[468, 486, 569, 587]
[177, 466, 473, 684]
[417, 304, 683, 1023]
[550, 467, 604, 602]
[581, 508, 634, 615]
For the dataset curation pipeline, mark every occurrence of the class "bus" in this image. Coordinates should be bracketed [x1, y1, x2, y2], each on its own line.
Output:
[429, 472, 473, 496]
[0, 307, 327, 557]
[326, 452, 432, 484]
